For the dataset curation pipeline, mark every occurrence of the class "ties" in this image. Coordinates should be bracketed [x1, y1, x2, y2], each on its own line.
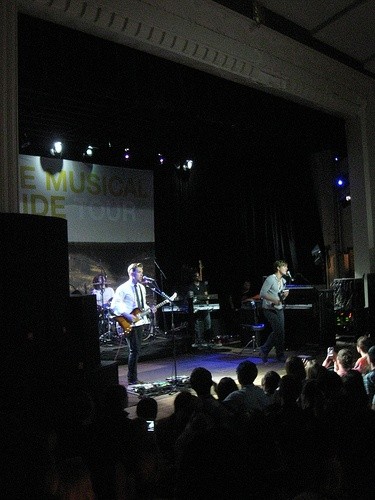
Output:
[133, 284, 141, 309]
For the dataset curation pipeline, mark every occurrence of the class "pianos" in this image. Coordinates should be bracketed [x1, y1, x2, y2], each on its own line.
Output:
[162, 303, 220, 313]
[282, 285, 337, 353]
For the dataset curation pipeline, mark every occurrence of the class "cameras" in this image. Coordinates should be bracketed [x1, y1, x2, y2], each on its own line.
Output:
[327, 347, 334, 359]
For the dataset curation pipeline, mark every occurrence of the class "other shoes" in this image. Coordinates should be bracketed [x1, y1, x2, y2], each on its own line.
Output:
[276, 354, 287, 362]
[257, 345, 267, 363]
[128, 380, 144, 385]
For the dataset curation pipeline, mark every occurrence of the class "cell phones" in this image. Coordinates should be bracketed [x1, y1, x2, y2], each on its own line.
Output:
[145, 420, 155, 433]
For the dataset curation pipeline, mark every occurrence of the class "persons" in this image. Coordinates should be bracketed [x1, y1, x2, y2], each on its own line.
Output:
[258, 258, 290, 363]
[0, 332, 375, 500]
[110, 262, 157, 386]
[90, 274, 115, 307]
[186, 272, 211, 345]
[228, 279, 261, 335]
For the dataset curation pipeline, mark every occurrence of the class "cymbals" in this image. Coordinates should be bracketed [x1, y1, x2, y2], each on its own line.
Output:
[92, 281, 117, 284]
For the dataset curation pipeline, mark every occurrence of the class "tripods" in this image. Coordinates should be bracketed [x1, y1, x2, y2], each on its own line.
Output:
[238, 305, 260, 354]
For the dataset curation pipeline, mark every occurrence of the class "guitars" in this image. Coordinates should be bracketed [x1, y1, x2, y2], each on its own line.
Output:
[113, 292, 178, 337]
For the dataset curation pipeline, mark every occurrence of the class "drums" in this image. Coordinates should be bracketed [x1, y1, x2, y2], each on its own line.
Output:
[141, 313, 153, 341]
[145, 294, 157, 307]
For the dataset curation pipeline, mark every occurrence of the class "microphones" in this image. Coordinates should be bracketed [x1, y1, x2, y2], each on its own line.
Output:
[143, 276, 155, 281]
[287, 271, 295, 281]
